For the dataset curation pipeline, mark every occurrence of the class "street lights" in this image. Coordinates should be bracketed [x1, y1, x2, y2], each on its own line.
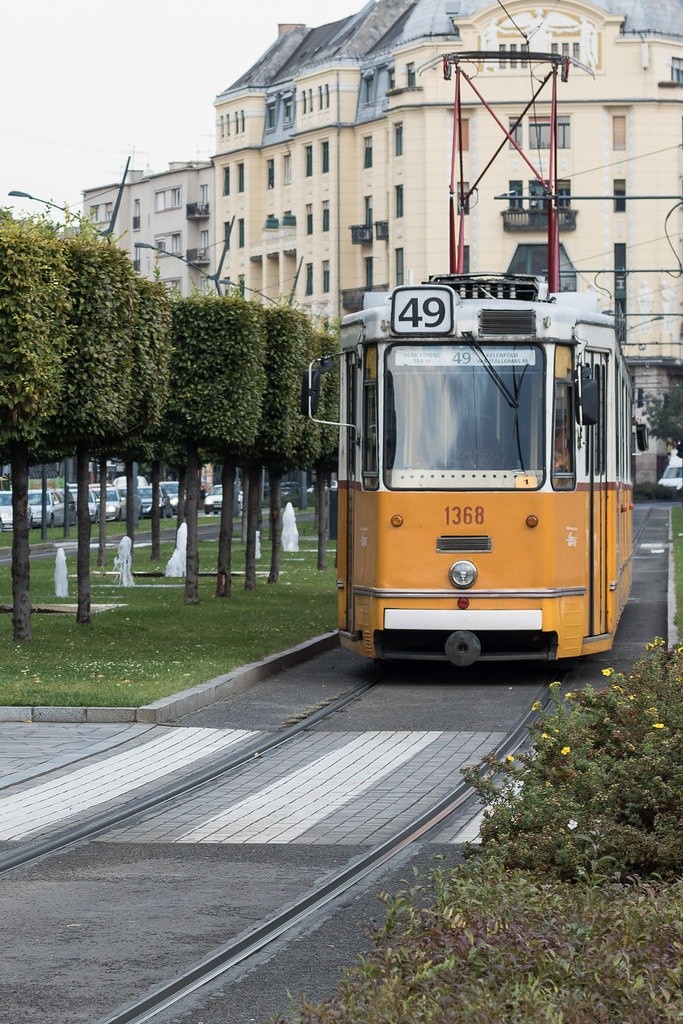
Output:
[134, 216, 236, 296]
[8, 156, 133, 244]
[218, 255, 307, 309]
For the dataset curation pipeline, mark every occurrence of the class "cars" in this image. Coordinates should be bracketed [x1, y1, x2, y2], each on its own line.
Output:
[657, 464, 683, 491]
[1, 480, 243, 530]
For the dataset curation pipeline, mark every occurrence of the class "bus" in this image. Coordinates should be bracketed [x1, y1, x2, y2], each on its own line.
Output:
[307, 272, 634, 680]
